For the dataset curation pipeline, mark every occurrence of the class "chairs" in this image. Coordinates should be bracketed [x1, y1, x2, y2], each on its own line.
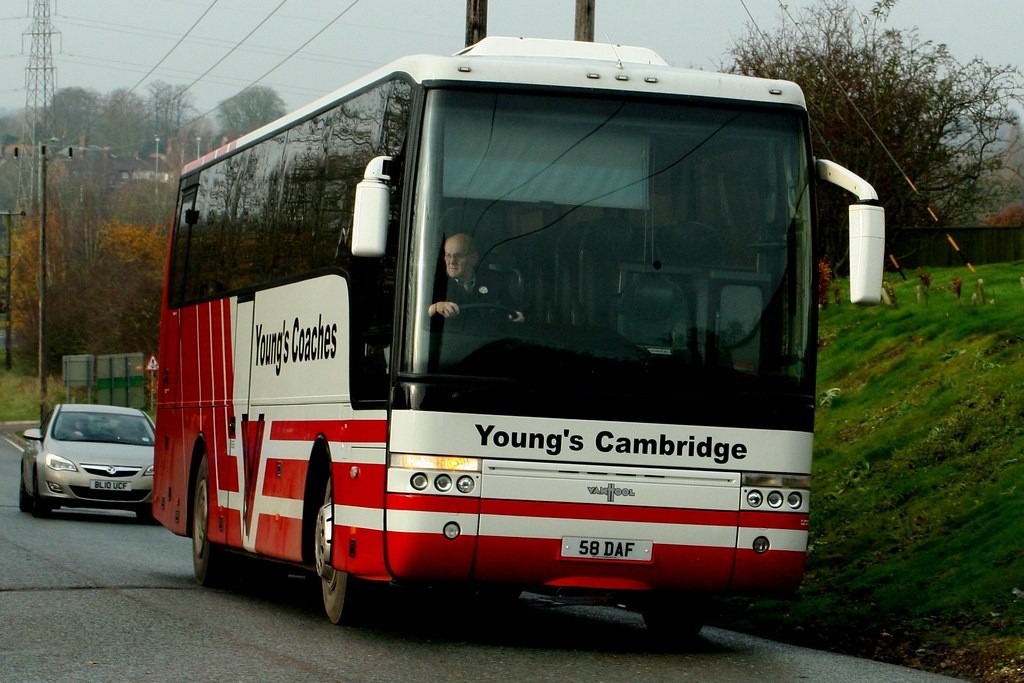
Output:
[617, 275, 688, 348]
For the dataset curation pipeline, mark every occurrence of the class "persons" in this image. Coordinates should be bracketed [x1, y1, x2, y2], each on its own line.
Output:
[428, 232, 526, 323]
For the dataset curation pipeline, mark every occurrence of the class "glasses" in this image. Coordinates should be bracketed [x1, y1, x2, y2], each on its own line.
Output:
[444, 251, 477, 262]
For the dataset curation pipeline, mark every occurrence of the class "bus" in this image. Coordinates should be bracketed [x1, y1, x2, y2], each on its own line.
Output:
[144, 35, 888, 648]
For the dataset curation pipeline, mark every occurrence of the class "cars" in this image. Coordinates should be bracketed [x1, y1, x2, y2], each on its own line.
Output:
[17, 403, 160, 526]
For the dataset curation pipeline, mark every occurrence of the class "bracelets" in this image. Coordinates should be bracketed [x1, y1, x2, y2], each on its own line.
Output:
[434, 302, 438, 312]
